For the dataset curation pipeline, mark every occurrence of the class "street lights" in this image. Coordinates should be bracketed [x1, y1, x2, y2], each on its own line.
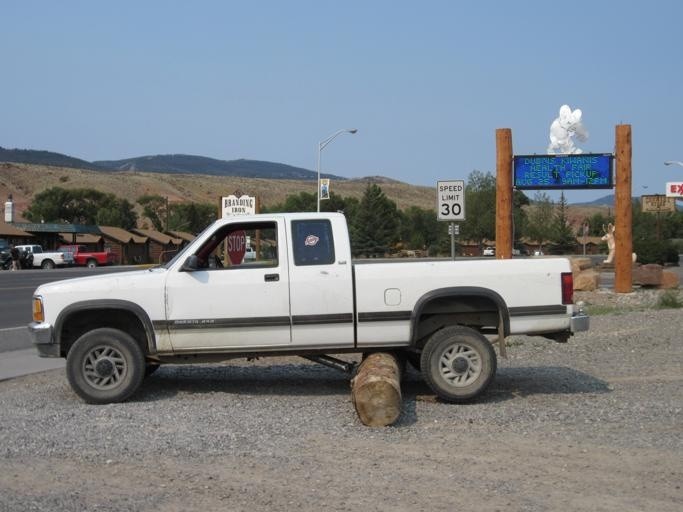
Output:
[317, 128, 357, 211]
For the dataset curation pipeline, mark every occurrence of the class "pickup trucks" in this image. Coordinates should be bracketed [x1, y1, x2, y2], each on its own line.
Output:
[30, 212, 588, 407]
[481, 247, 496, 256]
[14, 243, 120, 269]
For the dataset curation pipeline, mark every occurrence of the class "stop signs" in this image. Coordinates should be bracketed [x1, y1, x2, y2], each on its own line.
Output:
[226, 230, 247, 265]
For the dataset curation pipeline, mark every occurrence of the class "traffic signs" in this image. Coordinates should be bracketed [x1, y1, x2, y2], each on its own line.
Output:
[437, 182, 468, 220]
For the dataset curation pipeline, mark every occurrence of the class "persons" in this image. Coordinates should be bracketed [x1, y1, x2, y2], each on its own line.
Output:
[8, 243, 21, 271]
